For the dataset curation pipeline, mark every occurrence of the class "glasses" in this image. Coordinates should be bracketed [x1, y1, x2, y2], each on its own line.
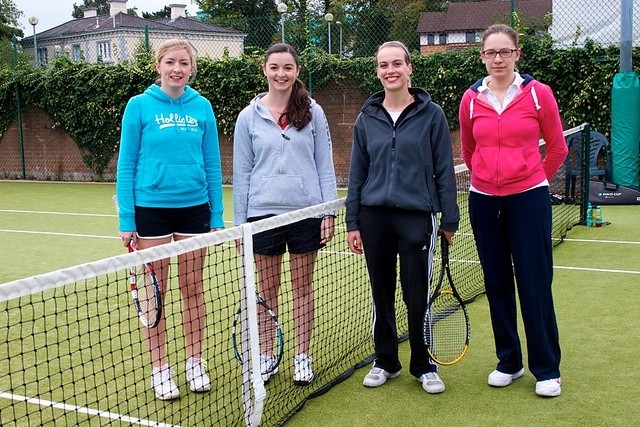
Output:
[482, 48, 518, 59]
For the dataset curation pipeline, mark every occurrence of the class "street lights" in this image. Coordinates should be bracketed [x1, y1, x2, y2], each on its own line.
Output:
[335, 20, 344, 56]
[55, 44, 72, 57]
[323, 12, 334, 53]
[28, 15, 38, 66]
[276, 2, 288, 43]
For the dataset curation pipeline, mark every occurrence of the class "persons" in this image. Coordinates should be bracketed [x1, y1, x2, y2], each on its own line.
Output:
[342, 39, 461, 396]
[457, 24, 570, 399]
[114, 37, 227, 402]
[231, 42, 340, 388]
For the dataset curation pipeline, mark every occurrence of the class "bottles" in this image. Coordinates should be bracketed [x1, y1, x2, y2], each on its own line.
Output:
[586, 205, 594, 226]
[595, 205, 602, 226]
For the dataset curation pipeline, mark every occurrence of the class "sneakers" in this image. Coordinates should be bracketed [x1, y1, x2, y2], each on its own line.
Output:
[416, 372, 445, 394]
[535, 377, 561, 397]
[151, 364, 181, 401]
[487, 367, 525, 387]
[293, 353, 315, 385]
[363, 367, 402, 387]
[249, 354, 279, 386]
[186, 357, 211, 392]
